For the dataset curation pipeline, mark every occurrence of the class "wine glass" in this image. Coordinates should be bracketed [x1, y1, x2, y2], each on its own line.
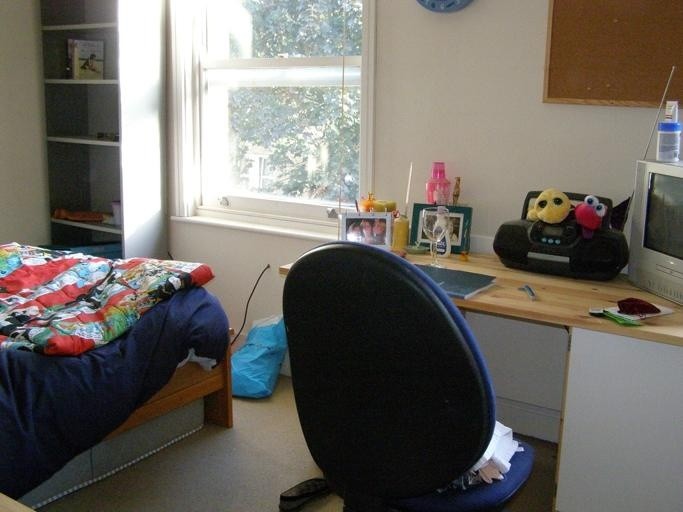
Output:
[422, 207, 450, 268]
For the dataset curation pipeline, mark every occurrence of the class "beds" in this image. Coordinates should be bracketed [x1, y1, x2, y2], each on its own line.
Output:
[0, 242, 234, 512]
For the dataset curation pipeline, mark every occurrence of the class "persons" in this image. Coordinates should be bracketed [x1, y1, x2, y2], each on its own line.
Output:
[346, 218, 386, 245]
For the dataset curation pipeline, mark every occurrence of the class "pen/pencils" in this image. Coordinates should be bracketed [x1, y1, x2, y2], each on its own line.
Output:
[524, 284, 535, 300]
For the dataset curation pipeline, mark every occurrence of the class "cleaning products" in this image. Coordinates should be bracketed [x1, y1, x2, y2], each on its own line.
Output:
[430, 205, 451, 256]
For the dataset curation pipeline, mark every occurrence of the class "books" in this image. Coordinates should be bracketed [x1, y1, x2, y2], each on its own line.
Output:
[415, 264, 497, 301]
[66, 38, 104, 80]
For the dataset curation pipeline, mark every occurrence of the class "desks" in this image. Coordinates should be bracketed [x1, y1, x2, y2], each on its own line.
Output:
[279, 249, 683, 371]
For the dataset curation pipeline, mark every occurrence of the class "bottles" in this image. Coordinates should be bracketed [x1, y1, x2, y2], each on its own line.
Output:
[425, 161, 451, 204]
[391, 214, 410, 253]
[655, 121, 681, 162]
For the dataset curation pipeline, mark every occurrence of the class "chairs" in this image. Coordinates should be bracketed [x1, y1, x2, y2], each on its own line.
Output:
[279, 240, 535, 512]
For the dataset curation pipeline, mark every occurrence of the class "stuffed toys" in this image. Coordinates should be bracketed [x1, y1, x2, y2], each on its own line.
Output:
[525, 188, 609, 230]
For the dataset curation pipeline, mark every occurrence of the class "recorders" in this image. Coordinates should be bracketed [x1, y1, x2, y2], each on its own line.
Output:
[492, 191, 628, 282]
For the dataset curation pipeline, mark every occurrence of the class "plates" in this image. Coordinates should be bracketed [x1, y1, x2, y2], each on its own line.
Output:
[409, 245, 429, 255]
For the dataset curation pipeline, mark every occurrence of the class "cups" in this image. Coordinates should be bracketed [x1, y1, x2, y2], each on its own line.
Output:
[360, 199, 372, 212]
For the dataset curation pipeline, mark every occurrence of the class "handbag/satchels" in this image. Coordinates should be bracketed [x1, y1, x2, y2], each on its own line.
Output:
[230, 324, 288, 399]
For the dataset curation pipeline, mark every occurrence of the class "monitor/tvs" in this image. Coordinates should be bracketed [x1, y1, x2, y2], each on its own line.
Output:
[627, 160, 683, 306]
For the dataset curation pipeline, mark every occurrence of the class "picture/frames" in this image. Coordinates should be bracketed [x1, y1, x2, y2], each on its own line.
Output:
[338, 212, 391, 251]
[410, 202, 472, 254]
[542, 0, 683, 109]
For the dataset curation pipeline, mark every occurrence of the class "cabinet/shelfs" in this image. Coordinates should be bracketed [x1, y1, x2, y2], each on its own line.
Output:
[43, 22, 122, 234]
